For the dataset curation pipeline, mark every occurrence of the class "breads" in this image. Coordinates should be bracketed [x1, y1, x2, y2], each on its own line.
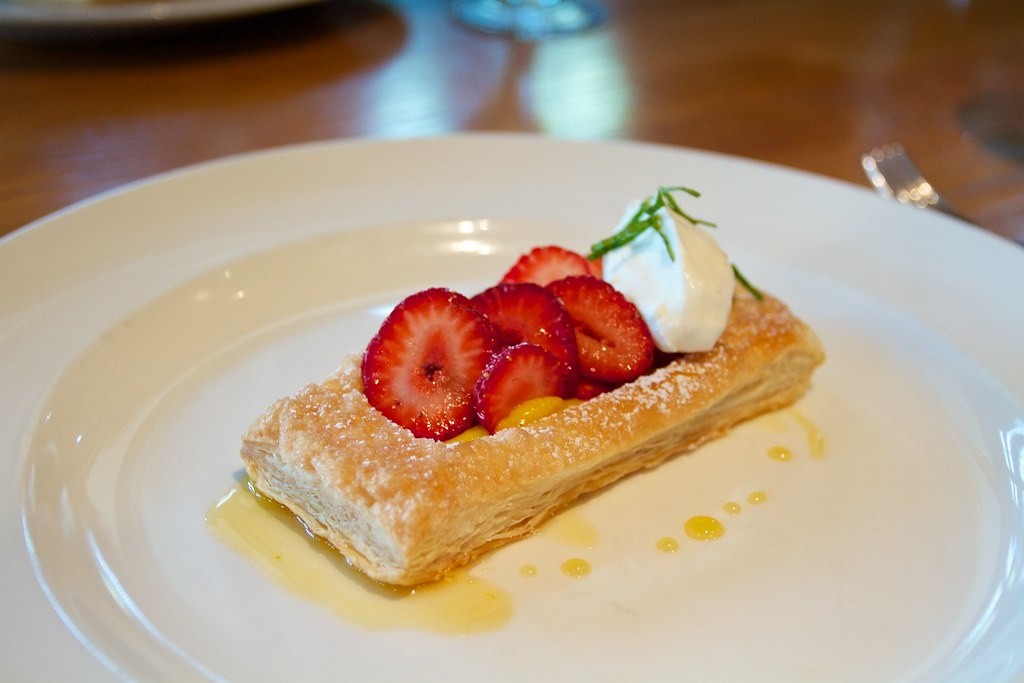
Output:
[241, 278, 825, 583]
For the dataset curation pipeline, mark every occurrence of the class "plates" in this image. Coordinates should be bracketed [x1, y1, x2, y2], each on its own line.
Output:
[0, 1, 306, 27]
[1, 130, 1024, 683]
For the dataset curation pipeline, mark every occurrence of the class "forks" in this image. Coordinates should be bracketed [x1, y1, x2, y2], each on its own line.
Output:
[858, 140, 984, 225]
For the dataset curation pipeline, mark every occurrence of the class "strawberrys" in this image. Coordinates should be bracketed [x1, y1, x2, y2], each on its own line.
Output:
[359, 246, 657, 442]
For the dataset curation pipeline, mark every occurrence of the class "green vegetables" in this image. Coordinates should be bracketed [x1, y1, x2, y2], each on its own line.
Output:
[586, 185, 765, 300]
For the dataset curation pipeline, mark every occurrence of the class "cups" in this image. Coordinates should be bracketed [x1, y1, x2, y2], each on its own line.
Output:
[452, 0, 613, 36]
